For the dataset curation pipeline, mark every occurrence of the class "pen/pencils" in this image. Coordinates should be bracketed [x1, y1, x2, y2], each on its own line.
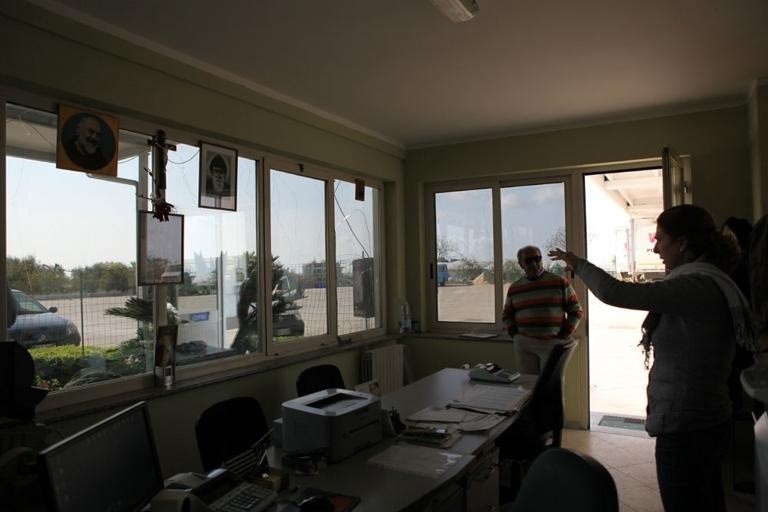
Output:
[391, 406, 399, 423]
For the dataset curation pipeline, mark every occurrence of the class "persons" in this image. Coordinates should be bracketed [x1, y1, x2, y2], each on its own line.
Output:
[548, 205, 734, 512]
[64, 115, 107, 172]
[502, 246, 585, 449]
[369, 383, 384, 397]
[236, 269, 258, 327]
[205, 152, 231, 196]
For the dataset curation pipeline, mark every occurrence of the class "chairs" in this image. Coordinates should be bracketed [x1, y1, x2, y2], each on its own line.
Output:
[493, 337, 578, 478]
[194, 395, 272, 482]
[296, 364, 346, 397]
[498, 447, 620, 512]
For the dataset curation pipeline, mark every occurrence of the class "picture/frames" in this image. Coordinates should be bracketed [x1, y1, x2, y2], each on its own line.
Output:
[354, 378, 379, 397]
[155, 325, 178, 387]
[137, 209, 184, 286]
[198, 140, 238, 212]
[355, 179, 365, 201]
[56, 103, 121, 177]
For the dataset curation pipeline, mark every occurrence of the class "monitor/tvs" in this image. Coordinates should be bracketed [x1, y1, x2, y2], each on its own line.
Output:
[39, 400, 165, 512]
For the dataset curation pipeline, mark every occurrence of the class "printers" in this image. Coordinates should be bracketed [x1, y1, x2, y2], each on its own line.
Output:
[281, 386, 382, 465]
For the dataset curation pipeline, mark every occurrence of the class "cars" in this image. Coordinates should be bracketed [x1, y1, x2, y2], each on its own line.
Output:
[5, 288, 81, 349]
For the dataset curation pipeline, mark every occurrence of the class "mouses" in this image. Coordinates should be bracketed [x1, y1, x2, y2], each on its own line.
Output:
[296, 493, 334, 509]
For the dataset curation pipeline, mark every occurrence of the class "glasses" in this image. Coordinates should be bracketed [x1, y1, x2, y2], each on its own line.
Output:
[212, 169, 226, 177]
[525, 256, 542, 265]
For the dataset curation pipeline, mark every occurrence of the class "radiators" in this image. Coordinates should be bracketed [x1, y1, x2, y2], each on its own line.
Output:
[362, 344, 404, 395]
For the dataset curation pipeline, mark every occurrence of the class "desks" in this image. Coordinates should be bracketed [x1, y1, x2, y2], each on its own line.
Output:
[136, 368, 539, 512]
